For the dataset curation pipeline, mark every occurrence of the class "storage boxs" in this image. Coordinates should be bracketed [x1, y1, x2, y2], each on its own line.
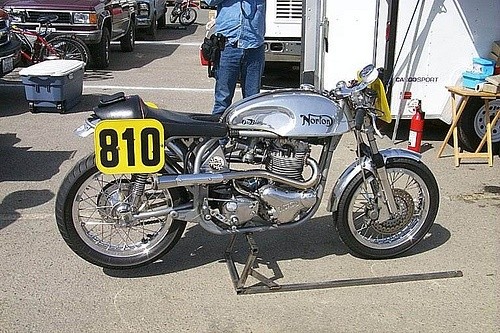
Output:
[19, 60, 86, 113]
[462, 41, 500, 94]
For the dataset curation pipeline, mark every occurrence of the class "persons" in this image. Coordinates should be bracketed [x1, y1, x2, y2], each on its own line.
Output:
[203, 0, 266, 116]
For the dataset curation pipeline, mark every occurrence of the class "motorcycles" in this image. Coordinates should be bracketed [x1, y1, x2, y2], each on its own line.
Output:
[56, 63, 440, 295]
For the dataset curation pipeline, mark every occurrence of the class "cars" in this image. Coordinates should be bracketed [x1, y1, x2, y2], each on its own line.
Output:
[0, 0, 138, 69]
[0, 10, 21, 79]
[138, 0, 167, 38]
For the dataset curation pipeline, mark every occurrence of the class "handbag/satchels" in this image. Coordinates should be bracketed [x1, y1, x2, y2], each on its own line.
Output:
[201, 38, 221, 61]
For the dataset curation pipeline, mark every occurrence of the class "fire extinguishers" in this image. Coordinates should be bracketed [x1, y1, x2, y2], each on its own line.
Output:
[408, 99, 426, 153]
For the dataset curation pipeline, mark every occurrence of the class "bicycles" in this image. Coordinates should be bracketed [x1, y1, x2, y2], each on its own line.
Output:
[0, 6, 92, 70]
[169, 0, 199, 25]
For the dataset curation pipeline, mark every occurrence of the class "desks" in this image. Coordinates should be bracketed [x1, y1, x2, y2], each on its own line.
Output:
[437, 86, 500, 167]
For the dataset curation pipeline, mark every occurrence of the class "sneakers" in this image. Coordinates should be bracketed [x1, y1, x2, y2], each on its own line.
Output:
[239, 151, 254, 162]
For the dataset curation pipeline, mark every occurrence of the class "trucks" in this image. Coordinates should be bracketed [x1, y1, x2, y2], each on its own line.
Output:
[302, 0, 500, 154]
